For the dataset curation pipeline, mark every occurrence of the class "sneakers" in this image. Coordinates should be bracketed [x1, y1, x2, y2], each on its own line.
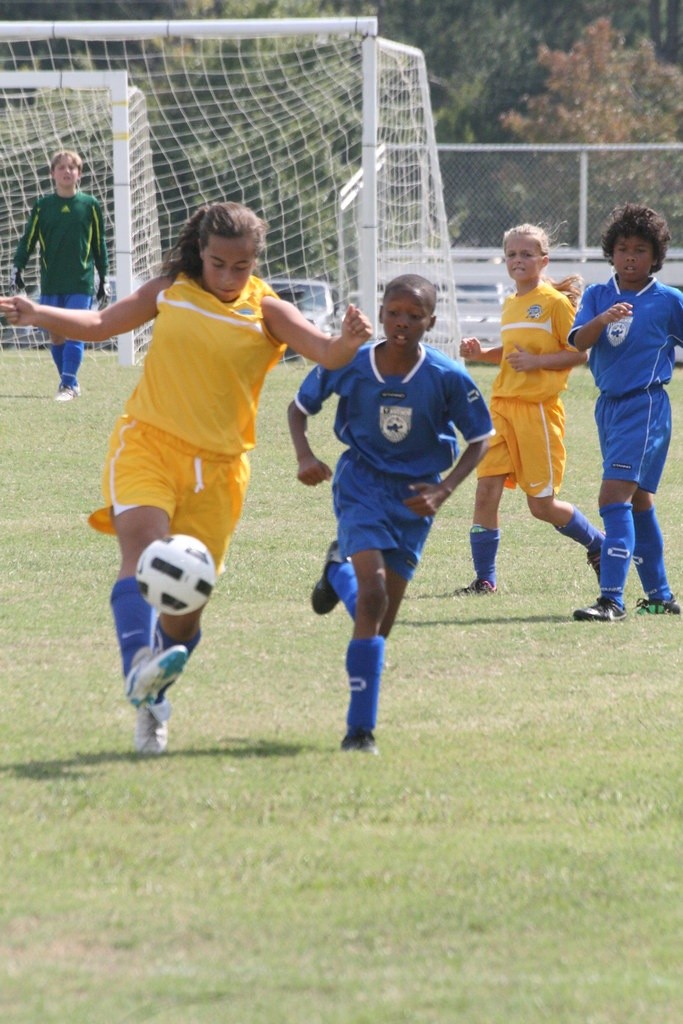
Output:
[53, 383, 81, 401]
[573, 598, 628, 622]
[458, 579, 498, 595]
[311, 540, 348, 615]
[587, 530, 606, 584]
[132, 697, 173, 756]
[124, 645, 189, 709]
[340, 730, 379, 756]
[635, 594, 680, 615]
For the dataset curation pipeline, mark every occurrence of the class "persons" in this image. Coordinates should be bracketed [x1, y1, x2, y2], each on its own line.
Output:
[451, 223, 612, 598]
[289, 274, 497, 752]
[10, 150, 112, 402]
[568, 204, 683, 621]
[0, 200, 374, 756]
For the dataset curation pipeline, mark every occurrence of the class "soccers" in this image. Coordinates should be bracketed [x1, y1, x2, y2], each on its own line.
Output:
[136, 534, 216, 616]
